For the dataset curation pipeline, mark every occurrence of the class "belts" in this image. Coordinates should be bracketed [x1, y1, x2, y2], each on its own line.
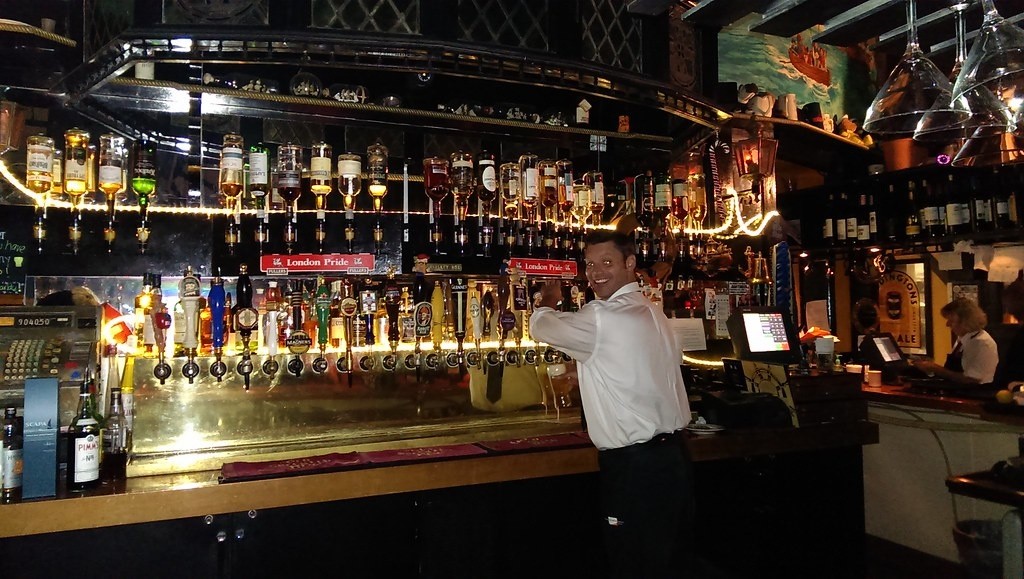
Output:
[601, 436, 675, 456]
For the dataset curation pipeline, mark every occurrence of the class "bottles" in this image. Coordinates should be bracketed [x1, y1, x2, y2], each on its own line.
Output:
[66, 379, 103, 493]
[424, 153, 604, 259]
[133, 274, 466, 390]
[0, 404, 22, 504]
[103, 353, 134, 446]
[821, 191, 882, 245]
[624, 171, 707, 265]
[104, 389, 128, 484]
[906, 173, 1024, 244]
[220, 130, 388, 256]
[26, 128, 157, 256]
[467, 276, 579, 375]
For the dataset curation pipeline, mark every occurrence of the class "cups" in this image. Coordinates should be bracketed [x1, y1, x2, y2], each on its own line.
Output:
[778, 94, 797, 120]
[546, 364, 573, 409]
[846, 364, 862, 373]
[749, 96, 773, 117]
[823, 114, 834, 132]
[868, 371, 881, 387]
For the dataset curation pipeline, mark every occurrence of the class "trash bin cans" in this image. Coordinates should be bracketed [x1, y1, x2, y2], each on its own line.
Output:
[952, 519, 1005, 578]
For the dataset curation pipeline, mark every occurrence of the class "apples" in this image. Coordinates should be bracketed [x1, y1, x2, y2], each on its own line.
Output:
[996, 390, 1013, 403]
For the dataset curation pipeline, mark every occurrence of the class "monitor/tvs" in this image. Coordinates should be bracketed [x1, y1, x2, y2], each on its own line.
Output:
[859, 332, 907, 370]
[725, 306, 802, 361]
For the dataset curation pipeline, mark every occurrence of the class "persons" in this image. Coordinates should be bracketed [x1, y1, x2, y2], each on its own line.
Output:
[529, 231, 692, 579]
[857, 303, 880, 360]
[910, 298, 999, 383]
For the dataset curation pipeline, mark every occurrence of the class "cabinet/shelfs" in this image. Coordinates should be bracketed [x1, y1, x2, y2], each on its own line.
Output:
[0, 88, 1024, 284]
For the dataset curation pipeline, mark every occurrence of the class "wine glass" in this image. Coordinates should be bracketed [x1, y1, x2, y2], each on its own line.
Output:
[863, 0, 1024, 166]
